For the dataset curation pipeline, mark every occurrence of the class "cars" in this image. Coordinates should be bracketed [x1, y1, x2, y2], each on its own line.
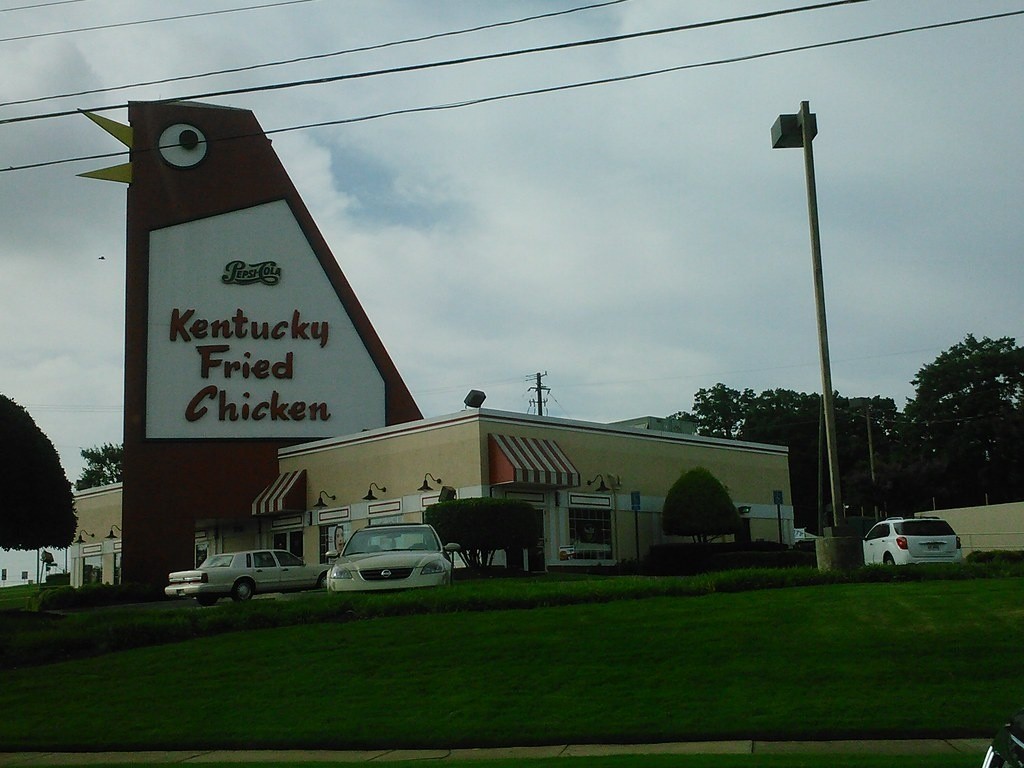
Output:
[326, 523, 460, 592]
[863, 516, 964, 567]
[164, 549, 333, 602]
